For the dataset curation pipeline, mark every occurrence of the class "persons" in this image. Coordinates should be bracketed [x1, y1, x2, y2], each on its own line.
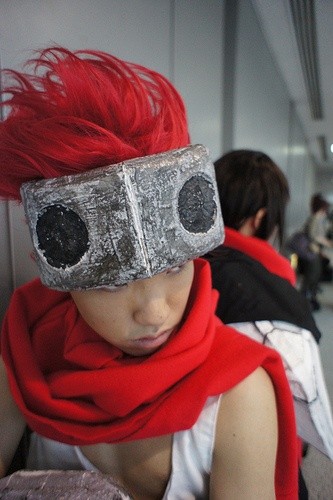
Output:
[0, 45, 298, 500]
[198, 149, 333, 500]
[299, 194, 333, 311]
